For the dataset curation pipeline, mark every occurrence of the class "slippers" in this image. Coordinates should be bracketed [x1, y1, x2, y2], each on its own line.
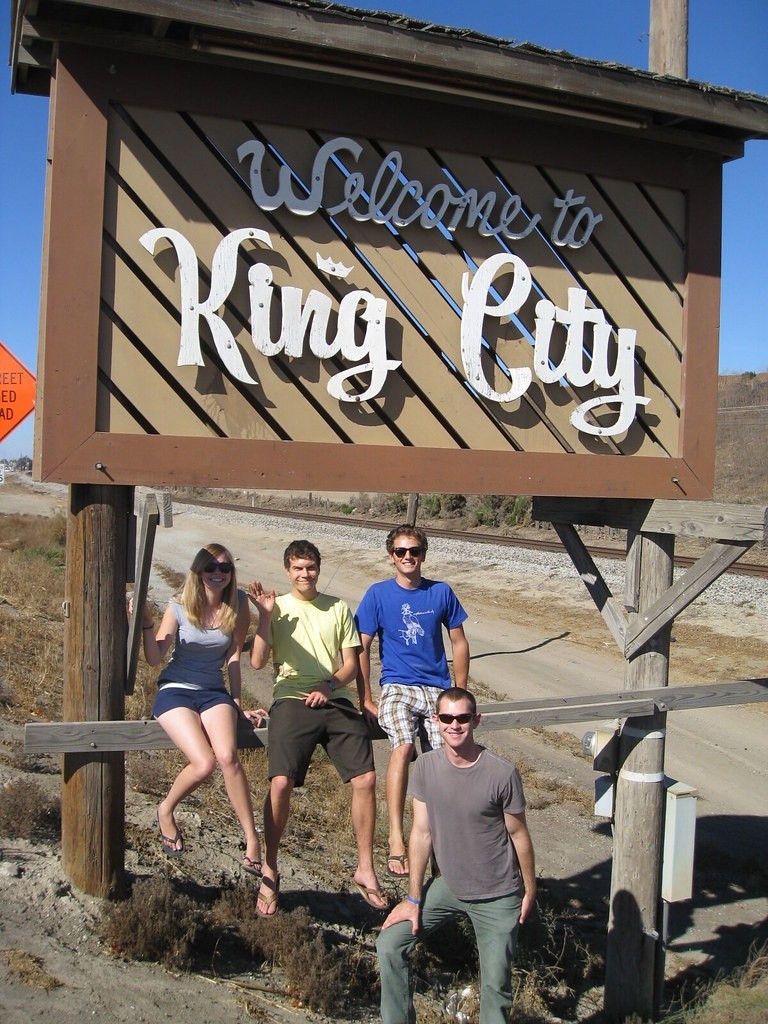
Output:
[352, 877, 391, 910]
[241, 851, 265, 877]
[386, 850, 410, 877]
[256, 874, 280, 918]
[156, 809, 185, 856]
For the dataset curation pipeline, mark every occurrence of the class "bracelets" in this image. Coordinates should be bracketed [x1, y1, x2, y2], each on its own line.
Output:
[142, 623, 155, 630]
[407, 896, 421, 905]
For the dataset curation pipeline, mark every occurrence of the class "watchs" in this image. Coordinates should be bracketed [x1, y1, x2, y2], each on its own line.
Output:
[324, 677, 336, 692]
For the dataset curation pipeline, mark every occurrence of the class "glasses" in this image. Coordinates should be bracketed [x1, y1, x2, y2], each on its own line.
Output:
[204, 562, 234, 573]
[438, 713, 475, 724]
[394, 546, 425, 558]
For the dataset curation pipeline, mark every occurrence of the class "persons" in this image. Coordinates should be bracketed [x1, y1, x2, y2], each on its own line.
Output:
[127, 543, 262, 878]
[376, 688, 535, 1024]
[249, 541, 391, 918]
[353, 524, 470, 877]
